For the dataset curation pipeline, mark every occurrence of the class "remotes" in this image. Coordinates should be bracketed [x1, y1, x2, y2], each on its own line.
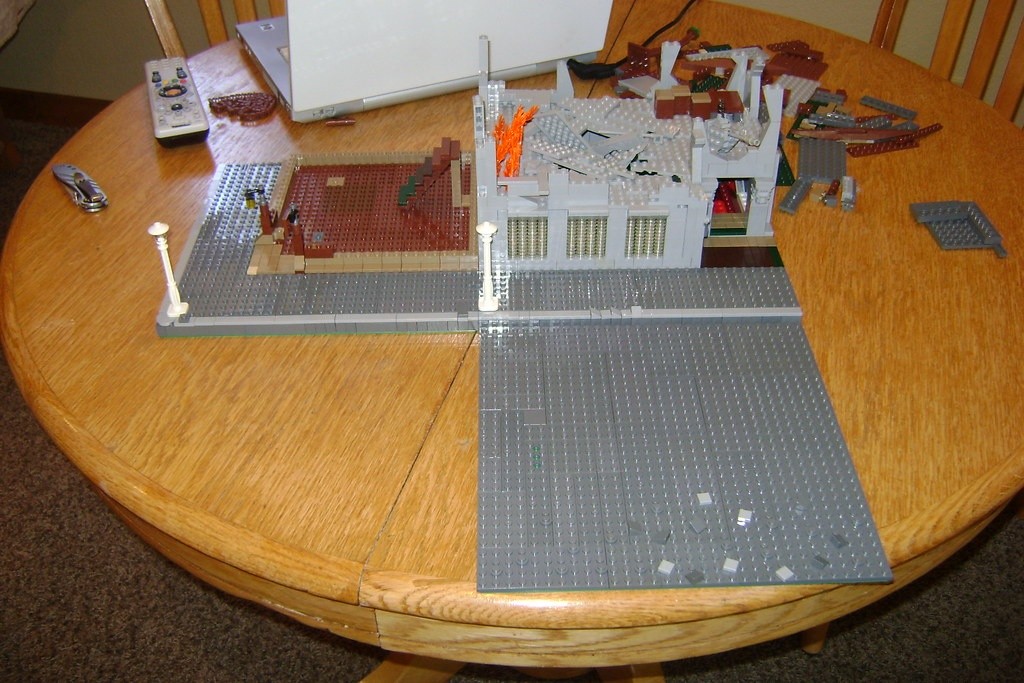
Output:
[145, 56, 210, 149]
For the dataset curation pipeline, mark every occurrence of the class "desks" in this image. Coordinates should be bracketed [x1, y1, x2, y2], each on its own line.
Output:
[0, 0, 1024, 683]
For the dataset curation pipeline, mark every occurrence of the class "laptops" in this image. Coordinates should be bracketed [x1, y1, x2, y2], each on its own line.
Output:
[236, 0, 613, 124]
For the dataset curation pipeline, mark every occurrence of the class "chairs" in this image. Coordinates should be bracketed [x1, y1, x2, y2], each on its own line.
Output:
[869, 0, 1024, 124]
[144, 0, 287, 59]
